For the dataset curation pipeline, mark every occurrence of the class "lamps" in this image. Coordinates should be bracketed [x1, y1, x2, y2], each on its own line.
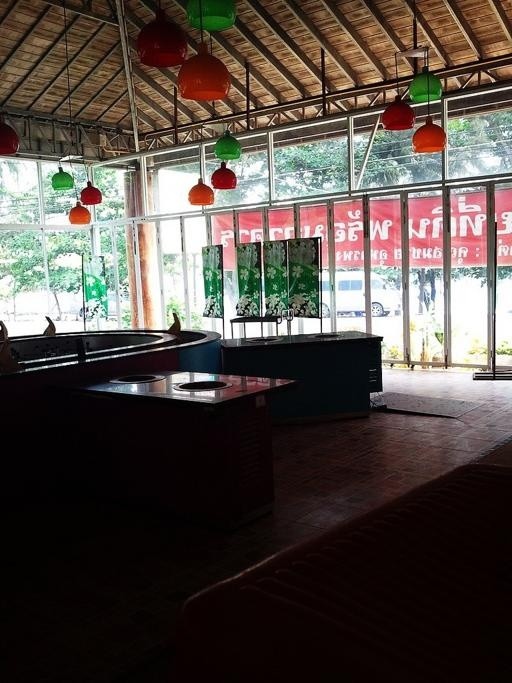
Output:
[188, 31, 241, 206]
[0, 114, 20, 155]
[52, 2, 102, 225]
[137, 1, 237, 101]
[382, 0, 446, 153]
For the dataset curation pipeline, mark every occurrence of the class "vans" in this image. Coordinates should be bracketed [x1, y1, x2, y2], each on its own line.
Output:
[319, 271, 398, 318]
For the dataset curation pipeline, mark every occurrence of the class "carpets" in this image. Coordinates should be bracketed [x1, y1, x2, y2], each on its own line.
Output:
[369, 391, 487, 419]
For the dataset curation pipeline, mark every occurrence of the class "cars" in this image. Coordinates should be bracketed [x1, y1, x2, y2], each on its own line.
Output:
[79, 284, 130, 318]
